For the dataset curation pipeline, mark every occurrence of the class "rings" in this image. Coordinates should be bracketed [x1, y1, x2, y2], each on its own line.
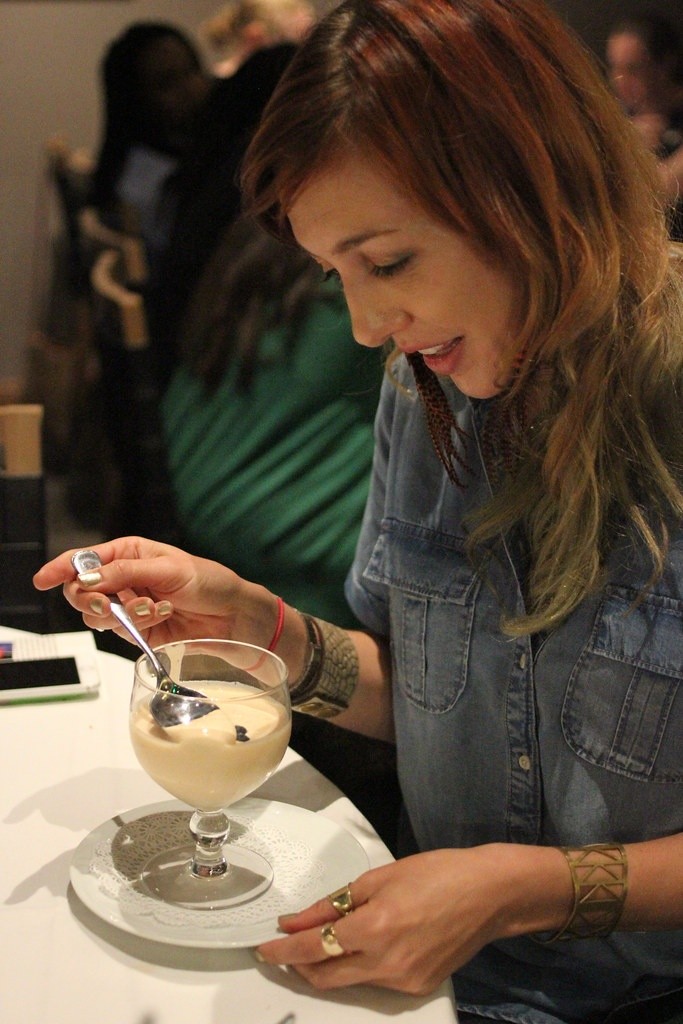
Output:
[319, 924, 344, 958]
[327, 880, 354, 914]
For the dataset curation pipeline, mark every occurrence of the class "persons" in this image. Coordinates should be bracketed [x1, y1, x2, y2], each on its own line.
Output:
[31, 0, 683, 1024]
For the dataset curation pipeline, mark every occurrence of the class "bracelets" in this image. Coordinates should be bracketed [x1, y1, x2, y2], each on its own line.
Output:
[554, 842, 629, 938]
[246, 595, 285, 672]
[288, 611, 360, 720]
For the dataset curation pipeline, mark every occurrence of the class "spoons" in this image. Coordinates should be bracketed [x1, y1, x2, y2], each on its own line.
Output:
[71, 549, 238, 746]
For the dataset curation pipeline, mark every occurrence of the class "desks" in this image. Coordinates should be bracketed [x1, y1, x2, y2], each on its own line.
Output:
[0, 626, 459, 1023]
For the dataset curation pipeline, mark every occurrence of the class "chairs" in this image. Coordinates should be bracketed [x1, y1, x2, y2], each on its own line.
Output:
[0, 141, 194, 662]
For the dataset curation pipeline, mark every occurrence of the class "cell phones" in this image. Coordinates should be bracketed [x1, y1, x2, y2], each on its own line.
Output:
[0, 654, 101, 705]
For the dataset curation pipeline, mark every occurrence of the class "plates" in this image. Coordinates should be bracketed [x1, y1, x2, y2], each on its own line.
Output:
[69, 797, 371, 949]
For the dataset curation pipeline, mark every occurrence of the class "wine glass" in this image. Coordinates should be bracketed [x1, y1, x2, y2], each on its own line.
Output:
[129, 639, 292, 910]
